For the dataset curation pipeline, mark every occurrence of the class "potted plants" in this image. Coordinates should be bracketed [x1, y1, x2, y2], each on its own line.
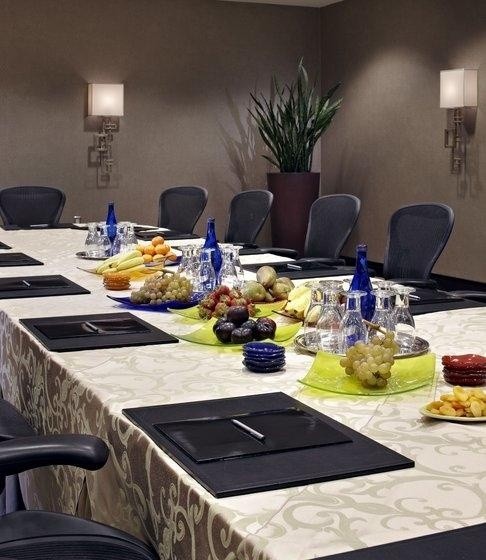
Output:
[246, 55, 344, 257]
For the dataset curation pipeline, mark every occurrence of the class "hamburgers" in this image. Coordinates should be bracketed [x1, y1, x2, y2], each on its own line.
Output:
[103, 272, 131, 289]
[442, 354, 486, 386]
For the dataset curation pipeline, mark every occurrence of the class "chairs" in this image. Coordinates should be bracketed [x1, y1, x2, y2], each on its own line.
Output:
[0, 396, 162, 560]
[225, 189, 274, 244]
[157, 185, 209, 235]
[0, 185, 66, 231]
[294, 193, 361, 266]
[384, 202, 486, 305]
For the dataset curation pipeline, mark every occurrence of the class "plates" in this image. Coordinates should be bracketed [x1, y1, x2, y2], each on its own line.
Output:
[241, 341, 289, 375]
[421, 402, 486, 424]
[293, 331, 437, 396]
[441, 353, 486, 388]
[104, 290, 304, 347]
[74, 255, 182, 290]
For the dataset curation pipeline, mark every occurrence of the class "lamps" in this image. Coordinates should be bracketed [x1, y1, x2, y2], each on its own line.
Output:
[440, 68, 478, 175]
[88, 83, 124, 175]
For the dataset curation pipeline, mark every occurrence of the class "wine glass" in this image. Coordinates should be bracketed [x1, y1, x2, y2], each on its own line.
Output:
[83, 219, 139, 260]
[300, 277, 417, 356]
[174, 238, 244, 295]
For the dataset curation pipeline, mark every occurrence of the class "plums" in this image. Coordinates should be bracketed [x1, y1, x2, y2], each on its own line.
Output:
[212, 305, 276, 344]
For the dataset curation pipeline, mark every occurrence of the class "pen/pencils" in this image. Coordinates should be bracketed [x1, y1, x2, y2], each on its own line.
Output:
[22, 280, 30, 287]
[407, 293, 422, 300]
[230, 418, 266, 441]
[84, 321, 99, 331]
[287, 263, 302, 270]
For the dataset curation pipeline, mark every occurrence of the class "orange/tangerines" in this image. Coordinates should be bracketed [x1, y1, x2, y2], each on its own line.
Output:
[137, 235, 177, 264]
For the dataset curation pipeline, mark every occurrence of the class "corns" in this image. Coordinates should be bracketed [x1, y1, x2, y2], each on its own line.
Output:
[96, 250, 145, 274]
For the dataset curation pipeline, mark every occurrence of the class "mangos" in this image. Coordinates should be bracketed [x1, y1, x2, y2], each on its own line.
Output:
[245, 265, 296, 302]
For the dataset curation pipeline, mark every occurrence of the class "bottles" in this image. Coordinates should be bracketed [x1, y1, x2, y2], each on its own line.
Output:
[102, 201, 121, 258]
[347, 243, 379, 346]
[199, 216, 225, 294]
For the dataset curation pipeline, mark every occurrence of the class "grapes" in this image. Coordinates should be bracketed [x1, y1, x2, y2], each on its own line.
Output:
[340, 331, 400, 388]
[131, 272, 194, 304]
